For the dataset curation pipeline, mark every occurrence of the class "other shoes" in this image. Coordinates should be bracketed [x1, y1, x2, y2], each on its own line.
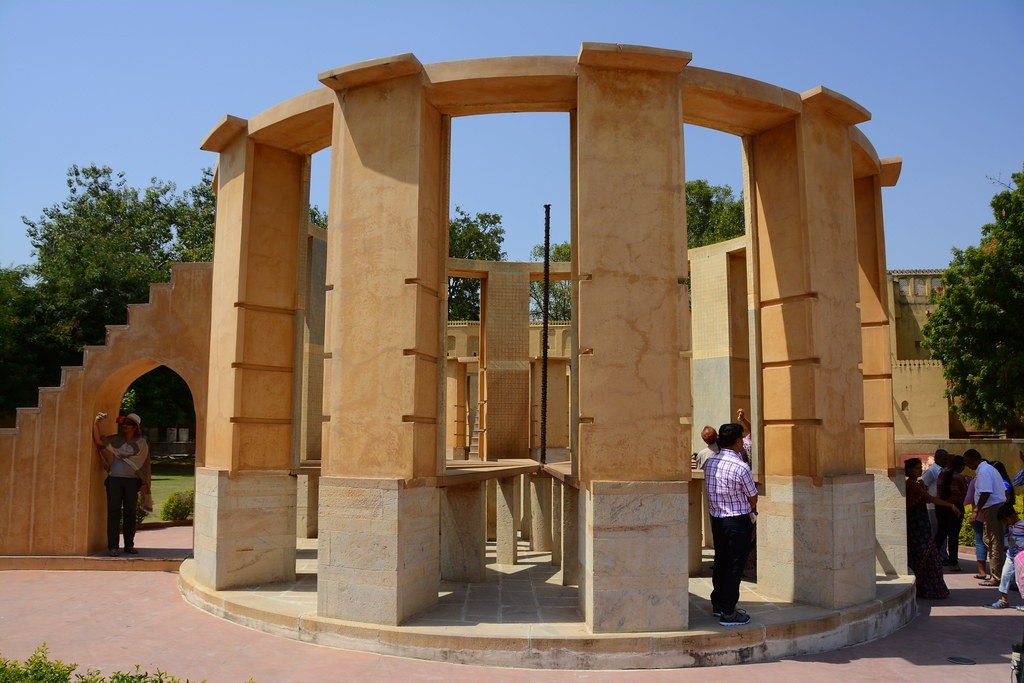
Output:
[978, 577, 1000, 585]
[950, 565, 967, 571]
[124, 546, 139, 555]
[975, 574, 986, 578]
[110, 549, 119, 556]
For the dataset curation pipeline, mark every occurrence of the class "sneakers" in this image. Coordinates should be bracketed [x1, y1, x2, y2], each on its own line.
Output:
[1016, 605, 1024, 610]
[720, 608, 750, 625]
[712, 608, 746, 616]
[992, 599, 1009, 608]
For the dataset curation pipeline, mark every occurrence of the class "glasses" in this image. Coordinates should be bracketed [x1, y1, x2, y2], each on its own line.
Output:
[122, 421, 135, 426]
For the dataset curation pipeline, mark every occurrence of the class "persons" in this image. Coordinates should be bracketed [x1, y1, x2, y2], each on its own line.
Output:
[697, 408, 759, 625]
[91, 410, 148, 557]
[903, 449, 1023, 609]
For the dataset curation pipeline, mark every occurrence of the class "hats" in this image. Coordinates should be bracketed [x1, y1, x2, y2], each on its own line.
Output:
[116, 413, 141, 437]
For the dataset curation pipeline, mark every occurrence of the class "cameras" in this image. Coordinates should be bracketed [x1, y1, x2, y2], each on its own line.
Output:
[100, 413, 107, 419]
